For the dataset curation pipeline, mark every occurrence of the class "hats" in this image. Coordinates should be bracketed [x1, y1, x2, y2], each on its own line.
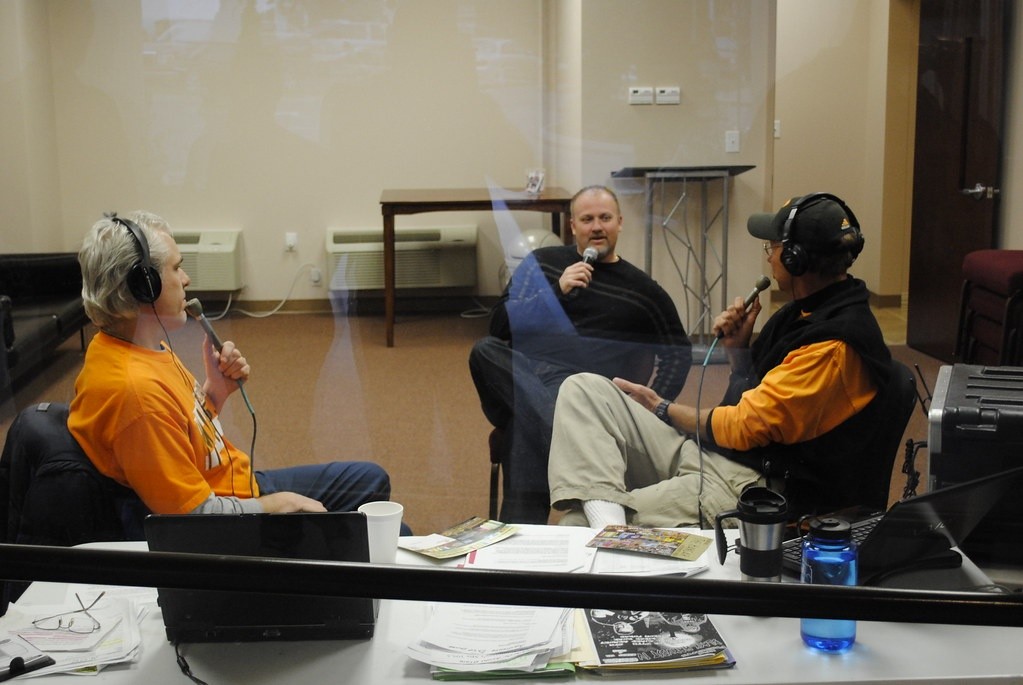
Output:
[747, 194, 863, 277]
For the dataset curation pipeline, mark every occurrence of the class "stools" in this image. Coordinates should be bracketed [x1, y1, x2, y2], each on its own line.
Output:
[954, 249, 1023, 365]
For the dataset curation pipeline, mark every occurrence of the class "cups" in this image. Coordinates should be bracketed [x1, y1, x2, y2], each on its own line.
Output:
[358, 501, 406, 564]
[716, 487, 788, 582]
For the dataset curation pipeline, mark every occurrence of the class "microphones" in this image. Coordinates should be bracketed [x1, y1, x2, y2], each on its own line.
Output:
[184, 298, 224, 354]
[717, 275, 771, 339]
[570, 248, 598, 300]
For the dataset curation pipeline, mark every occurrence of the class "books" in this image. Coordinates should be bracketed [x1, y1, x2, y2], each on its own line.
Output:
[435, 608, 739, 677]
[0, 634, 56, 682]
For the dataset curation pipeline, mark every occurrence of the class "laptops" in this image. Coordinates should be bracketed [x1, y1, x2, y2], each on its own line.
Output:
[781, 468, 1023, 577]
[145, 511, 377, 644]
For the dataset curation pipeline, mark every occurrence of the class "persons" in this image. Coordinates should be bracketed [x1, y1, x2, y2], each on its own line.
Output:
[469, 187, 691, 525]
[68, 210, 413, 536]
[548, 192, 895, 526]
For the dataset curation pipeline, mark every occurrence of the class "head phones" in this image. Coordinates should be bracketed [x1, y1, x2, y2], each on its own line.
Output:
[780, 191, 865, 276]
[111, 218, 162, 303]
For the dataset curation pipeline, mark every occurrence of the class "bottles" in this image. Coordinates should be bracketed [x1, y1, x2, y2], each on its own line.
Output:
[796, 514, 857, 648]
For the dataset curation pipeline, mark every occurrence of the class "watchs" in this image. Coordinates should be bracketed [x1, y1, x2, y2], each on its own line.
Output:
[654, 399, 672, 426]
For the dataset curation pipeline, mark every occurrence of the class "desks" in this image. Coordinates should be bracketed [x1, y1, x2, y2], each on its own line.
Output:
[612, 162, 756, 347]
[0, 519, 1023, 685]
[376, 186, 573, 346]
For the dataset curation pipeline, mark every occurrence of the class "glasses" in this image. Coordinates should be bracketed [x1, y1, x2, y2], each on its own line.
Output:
[31, 591, 105, 634]
[762, 242, 786, 255]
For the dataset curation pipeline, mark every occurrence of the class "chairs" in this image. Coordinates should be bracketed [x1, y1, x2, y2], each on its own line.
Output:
[759, 360, 922, 520]
[0, 406, 135, 605]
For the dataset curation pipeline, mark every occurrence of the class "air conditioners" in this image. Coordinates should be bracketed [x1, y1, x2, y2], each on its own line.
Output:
[328, 223, 479, 291]
[167, 230, 245, 294]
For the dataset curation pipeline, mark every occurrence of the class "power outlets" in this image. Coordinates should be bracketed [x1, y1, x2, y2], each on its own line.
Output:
[287, 232, 299, 251]
[311, 268, 321, 286]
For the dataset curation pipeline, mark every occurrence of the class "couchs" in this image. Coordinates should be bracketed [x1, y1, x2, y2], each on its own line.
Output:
[0, 252, 94, 403]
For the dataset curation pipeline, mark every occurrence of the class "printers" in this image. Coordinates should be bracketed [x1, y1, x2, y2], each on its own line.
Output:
[928, 362, 1023, 495]
